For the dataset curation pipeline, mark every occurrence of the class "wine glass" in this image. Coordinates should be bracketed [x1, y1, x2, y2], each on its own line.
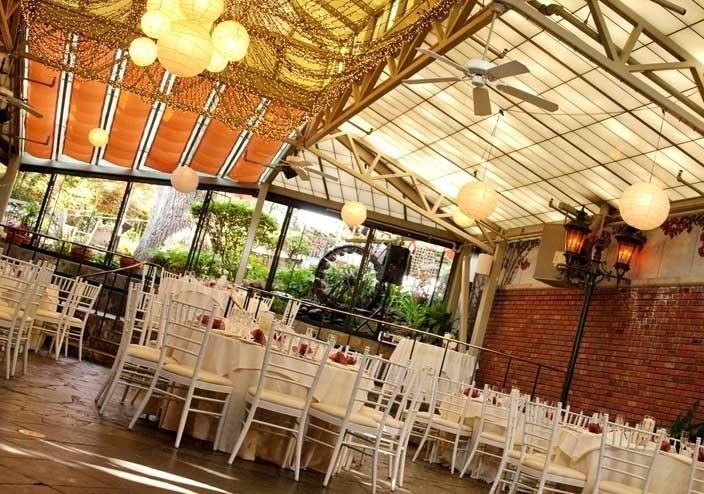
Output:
[679, 431, 688, 454]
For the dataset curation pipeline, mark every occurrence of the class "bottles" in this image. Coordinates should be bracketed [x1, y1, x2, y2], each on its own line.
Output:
[614, 413, 671, 447]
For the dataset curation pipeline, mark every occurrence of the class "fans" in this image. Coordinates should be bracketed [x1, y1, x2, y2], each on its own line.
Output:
[256, 146, 338, 182]
[401, 2, 558, 116]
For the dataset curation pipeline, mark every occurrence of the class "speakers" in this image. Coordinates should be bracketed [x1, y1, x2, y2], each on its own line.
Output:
[532, 222, 572, 288]
[376, 244, 411, 286]
[475, 253, 493, 277]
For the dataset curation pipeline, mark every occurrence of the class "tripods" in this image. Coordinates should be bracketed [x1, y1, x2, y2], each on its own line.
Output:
[353, 284, 392, 340]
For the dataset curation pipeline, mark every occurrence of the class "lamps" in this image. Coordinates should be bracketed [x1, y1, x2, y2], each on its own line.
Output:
[88, 128, 108, 147]
[453, 116, 501, 228]
[619, 108, 670, 230]
[129, 0, 250, 78]
[341, 182, 367, 226]
[171, 165, 200, 193]
